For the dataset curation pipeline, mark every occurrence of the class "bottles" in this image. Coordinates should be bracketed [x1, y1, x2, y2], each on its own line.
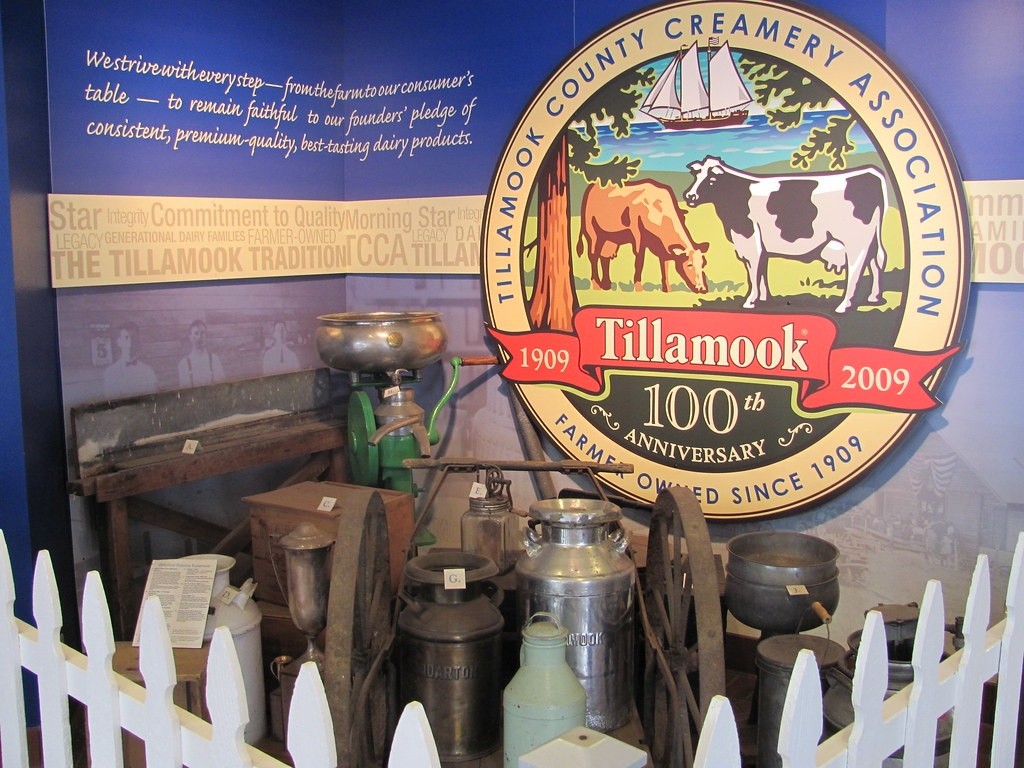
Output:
[460, 495, 520, 575]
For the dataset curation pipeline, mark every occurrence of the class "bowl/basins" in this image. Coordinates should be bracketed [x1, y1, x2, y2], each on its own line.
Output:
[316, 312, 445, 382]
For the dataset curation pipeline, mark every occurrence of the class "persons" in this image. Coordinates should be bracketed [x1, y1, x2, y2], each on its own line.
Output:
[104, 321, 157, 399]
[177, 319, 225, 390]
[262, 319, 301, 374]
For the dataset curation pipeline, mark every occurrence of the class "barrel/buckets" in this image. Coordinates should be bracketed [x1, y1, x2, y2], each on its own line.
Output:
[396, 554, 505, 762]
[823, 628, 953, 759]
[179, 554, 265, 744]
[517, 500, 635, 734]
[502, 611, 586, 768]
[753, 602, 847, 768]
